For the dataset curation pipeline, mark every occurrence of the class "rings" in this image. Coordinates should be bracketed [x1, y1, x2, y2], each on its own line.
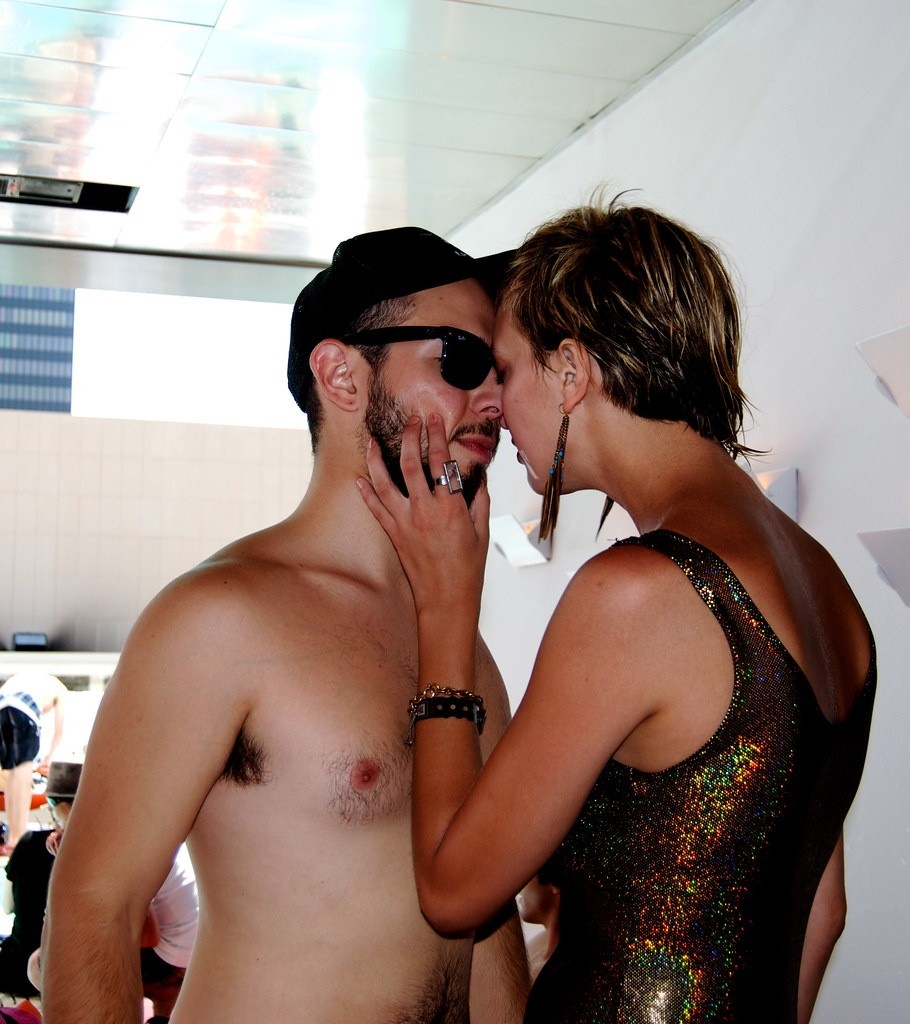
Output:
[437, 459, 464, 494]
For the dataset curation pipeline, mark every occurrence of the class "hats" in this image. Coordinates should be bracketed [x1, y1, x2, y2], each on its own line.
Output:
[288, 226, 518, 412]
[43, 761, 83, 798]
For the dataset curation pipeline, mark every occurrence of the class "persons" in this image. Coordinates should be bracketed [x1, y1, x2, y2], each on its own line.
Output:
[357, 206, 877, 1024]
[518, 845, 563, 981]
[42, 228, 533, 1024]
[0, 675, 63, 853]
[0, 761, 200, 1023]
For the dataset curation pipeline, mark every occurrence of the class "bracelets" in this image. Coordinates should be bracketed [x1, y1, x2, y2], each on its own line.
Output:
[405, 684, 485, 745]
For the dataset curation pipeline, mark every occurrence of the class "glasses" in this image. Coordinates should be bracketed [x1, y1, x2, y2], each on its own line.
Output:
[47, 796, 64, 807]
[340, 326, 496, 391]
